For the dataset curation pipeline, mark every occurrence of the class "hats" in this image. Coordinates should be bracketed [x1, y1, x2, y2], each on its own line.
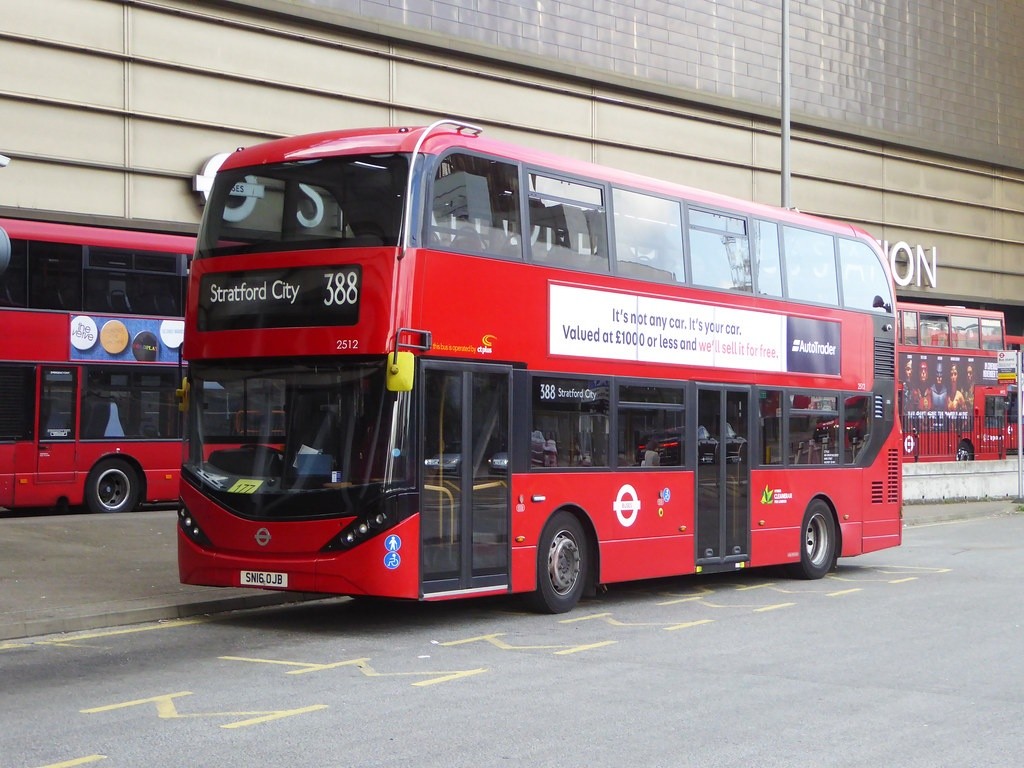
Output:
[646, 440, 658, 450]
[698, 425, 709, 440]
[543, 439, 557, 452]
[725, 422, 737, 438]
[913, 428, 916, 431]
[531, 430, 546, 442]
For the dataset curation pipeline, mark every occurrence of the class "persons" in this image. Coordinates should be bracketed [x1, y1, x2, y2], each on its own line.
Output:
[902, 358, 977, 431]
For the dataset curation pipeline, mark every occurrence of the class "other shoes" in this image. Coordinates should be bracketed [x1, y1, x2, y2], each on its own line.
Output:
[915, 458, 917, 462]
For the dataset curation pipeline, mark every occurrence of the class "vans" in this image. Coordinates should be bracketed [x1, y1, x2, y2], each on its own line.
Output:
[813, 403, 835, 442]
[833, 395, 869, 443]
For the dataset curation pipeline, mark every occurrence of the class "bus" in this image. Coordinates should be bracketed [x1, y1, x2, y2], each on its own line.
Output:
[898, 329, 1024, 455]
[0, 216, 289, 514]
[894, 299, 1008, 463]
[174, 121, 903, 615]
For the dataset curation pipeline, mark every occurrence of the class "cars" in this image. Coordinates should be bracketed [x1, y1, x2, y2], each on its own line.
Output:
[582, 450, 591, 465]
[635, 426, 683, 466]
[486, 441, 509, 474]
[425, 442, 464, 475]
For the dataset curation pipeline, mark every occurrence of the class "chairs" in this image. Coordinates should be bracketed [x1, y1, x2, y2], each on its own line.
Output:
[5, 285, 163, 315]
